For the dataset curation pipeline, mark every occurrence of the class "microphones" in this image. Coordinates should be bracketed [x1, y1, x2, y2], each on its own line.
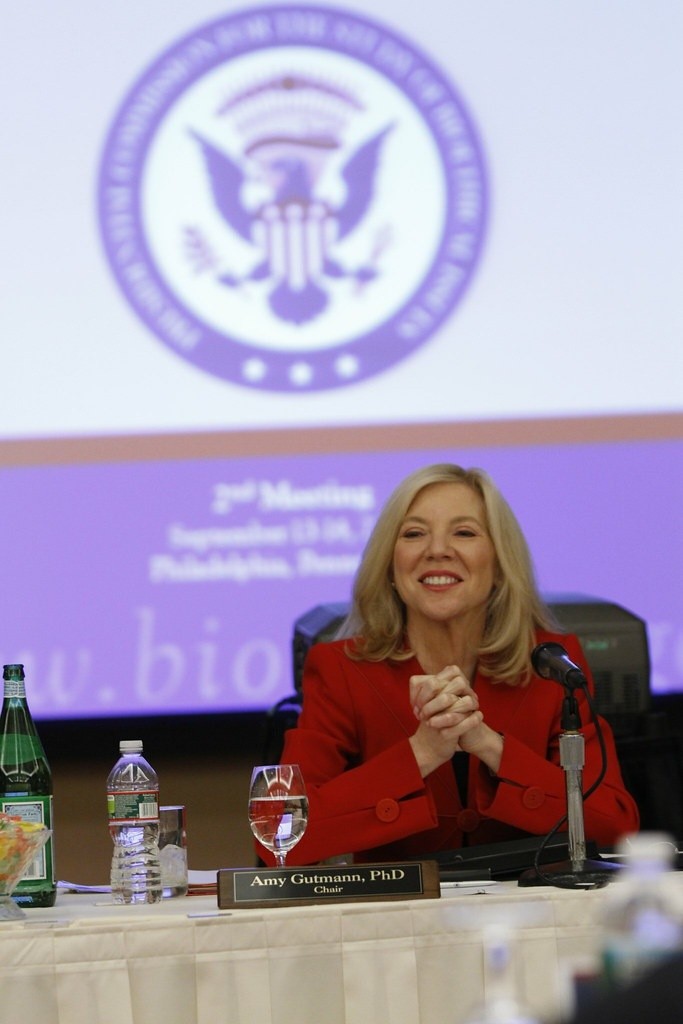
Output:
[531, 642, 588, 690]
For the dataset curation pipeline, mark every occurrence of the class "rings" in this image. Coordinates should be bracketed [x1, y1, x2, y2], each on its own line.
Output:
[456, 695, 462, 700]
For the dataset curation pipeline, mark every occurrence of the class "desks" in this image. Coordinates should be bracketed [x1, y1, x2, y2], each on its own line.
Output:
[0, 867, 683, 1024]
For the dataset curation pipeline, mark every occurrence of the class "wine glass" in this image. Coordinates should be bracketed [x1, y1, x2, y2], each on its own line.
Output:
[246, 764, 311, 867]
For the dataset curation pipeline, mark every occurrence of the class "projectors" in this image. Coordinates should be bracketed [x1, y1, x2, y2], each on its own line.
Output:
[293, 590, 651, 740]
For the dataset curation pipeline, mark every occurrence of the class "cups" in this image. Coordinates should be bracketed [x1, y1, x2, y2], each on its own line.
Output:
[160, 805, 188, 895]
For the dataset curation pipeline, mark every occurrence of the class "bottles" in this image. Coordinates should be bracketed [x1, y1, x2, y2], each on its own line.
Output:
[105, 741, 160, 902]
[0, 661, 58, 909]
[587, 830, 683, 991]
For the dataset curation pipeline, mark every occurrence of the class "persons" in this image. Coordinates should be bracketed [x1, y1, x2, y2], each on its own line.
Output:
[254, 463, 639, 870]
